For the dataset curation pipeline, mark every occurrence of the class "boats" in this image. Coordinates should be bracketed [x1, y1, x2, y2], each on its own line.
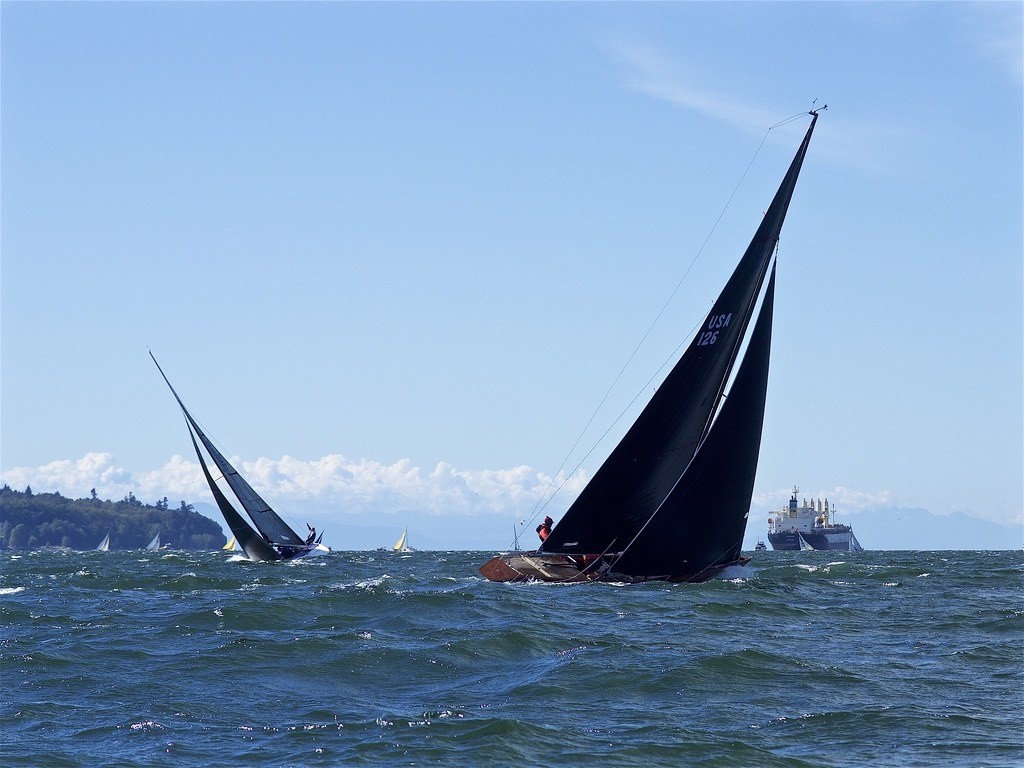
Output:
[766, 485, 862, 552]
[755, 540, 767, 552]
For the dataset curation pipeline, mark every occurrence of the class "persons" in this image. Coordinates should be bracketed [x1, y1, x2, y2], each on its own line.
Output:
[536, 516, 554, 542]
[305, 522, 316, 546]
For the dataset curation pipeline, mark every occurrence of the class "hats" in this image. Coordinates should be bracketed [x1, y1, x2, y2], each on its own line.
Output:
[544, 516, 554, 524]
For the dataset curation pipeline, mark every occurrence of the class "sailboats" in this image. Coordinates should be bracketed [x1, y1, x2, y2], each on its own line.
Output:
[797, 531, 815, 552]
[96, 531, 111, 552]
[144, 342, 327, 563]
[222, 535, 236, 553]
[474, 103, 832, 586]
[146, 530, 161, 551]
[391, 525, 409, 553]
